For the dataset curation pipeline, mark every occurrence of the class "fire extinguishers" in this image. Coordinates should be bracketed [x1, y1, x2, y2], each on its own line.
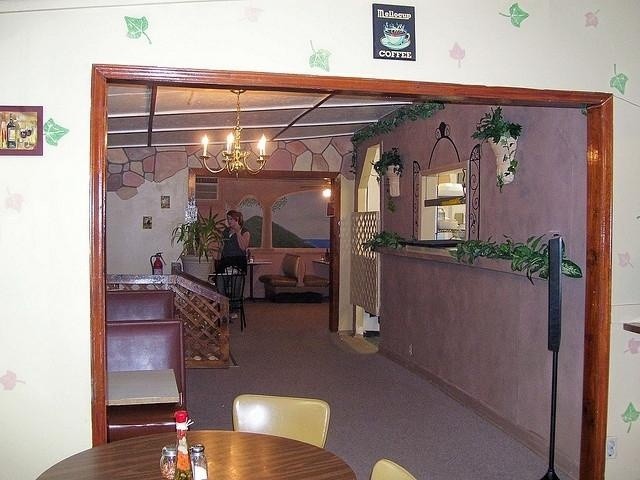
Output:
[150, 252, 167, 274]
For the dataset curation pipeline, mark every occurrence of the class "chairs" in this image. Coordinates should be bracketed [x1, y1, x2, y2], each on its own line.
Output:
[211, 265, 249, 332]
[368, 455, 420, 479]
[228, 392, 332, 450]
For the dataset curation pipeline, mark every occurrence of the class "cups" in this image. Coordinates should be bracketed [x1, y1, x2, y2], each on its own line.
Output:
[384, 29, 410, 45]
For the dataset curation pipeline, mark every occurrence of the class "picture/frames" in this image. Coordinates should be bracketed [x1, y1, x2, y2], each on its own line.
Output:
[0, 105, 44, 156]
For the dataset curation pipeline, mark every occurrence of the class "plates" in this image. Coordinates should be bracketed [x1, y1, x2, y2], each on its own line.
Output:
[380, 37, 411, 50]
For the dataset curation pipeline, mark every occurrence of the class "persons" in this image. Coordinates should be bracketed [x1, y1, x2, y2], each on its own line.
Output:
[208, 210, 251, 321]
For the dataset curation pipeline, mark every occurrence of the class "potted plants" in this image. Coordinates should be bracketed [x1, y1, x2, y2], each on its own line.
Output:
[470, 103, 524, 195]
[368, 142, 405, 214]
[170, 203, 232, 284]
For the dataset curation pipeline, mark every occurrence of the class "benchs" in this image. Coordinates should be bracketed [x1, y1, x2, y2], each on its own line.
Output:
[300, 256, 332, 305]
[258, 253, 300, 304]
[106, 317, 192, 445]
[105, 289, 179, 324]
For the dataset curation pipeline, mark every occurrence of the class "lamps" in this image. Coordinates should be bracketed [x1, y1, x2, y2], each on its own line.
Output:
[197, 87, 275, 181]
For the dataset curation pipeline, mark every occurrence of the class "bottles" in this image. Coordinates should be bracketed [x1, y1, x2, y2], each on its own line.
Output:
[6, 113, 16, 149]
[174, 410, 193, 480]
[160, 444, 177, 480]
[190, 442, 207, 480]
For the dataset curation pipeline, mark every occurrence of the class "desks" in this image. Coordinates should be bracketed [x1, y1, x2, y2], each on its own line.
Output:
[105, 367, 181, 408]
[241, 259, 277, 306]
[311, 258, 330, 266]
[205, 271, 248, 326]
[35, 425, 360, 480]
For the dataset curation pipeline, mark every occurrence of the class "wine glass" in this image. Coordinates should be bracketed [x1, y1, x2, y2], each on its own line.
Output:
[20, 127, 34, 148]
